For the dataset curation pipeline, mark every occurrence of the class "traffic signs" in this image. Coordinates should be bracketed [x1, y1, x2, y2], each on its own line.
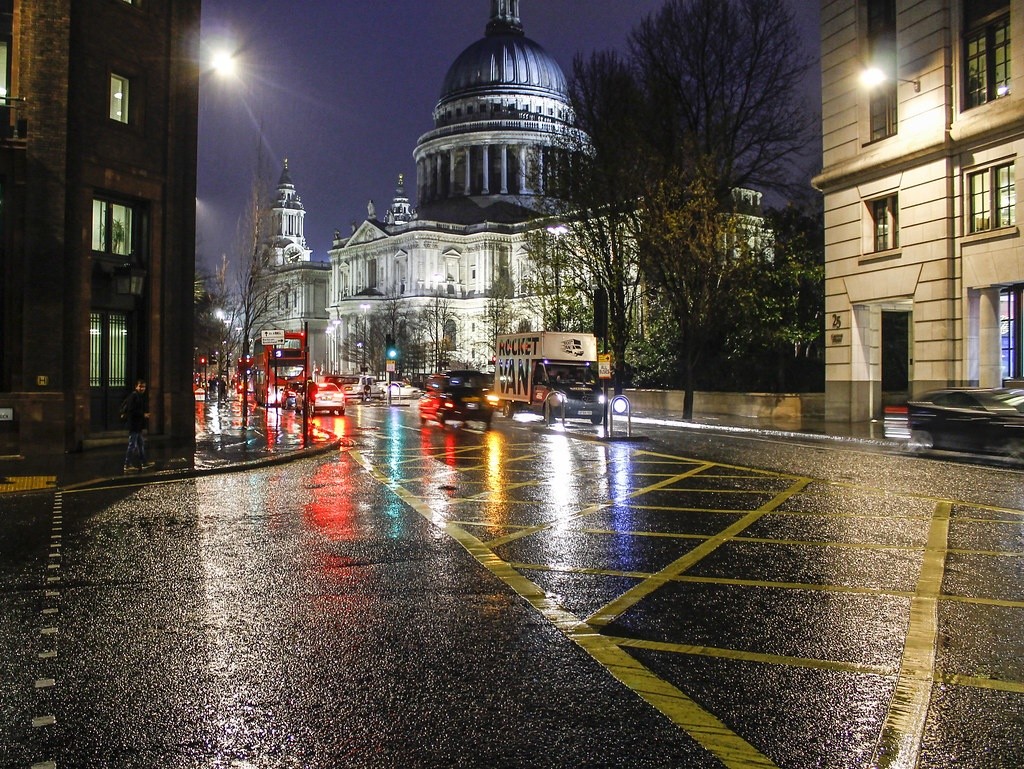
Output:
[261, 328, 285, 346]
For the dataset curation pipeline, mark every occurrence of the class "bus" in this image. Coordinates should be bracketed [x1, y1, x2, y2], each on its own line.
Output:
[237, 357, 254, 394]
[253, 332, 310, 405]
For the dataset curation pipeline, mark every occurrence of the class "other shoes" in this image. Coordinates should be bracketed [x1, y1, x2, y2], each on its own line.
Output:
[141, 462, 156, 470]
[124, 464, 141, 470]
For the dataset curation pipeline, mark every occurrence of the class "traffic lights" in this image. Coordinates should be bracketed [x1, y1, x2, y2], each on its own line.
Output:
[202, 357, 204, 363]
[386, 339, 397, 360]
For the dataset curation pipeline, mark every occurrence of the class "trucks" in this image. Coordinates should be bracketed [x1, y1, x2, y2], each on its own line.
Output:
[495, 330, 605, 424]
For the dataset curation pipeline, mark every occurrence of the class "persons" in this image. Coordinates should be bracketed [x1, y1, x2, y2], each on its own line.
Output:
[208, 377, 226, 393]
[122, 379, 155, 471]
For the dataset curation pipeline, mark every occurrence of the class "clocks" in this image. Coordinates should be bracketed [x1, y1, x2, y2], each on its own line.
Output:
[284, 246, 302, 264]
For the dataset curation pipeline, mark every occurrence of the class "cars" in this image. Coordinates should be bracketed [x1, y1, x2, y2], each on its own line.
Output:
[418, 369, 493, 431]
[324, 375, 386, 402]
[906, 386, 1024, 459]
[379, 380, 422, 400]
[279, 381, 306, 410]
[295, 382, 346, 416]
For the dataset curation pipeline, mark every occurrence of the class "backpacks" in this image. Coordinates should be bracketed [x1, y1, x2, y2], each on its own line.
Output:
[118, 390, 140, 424]
